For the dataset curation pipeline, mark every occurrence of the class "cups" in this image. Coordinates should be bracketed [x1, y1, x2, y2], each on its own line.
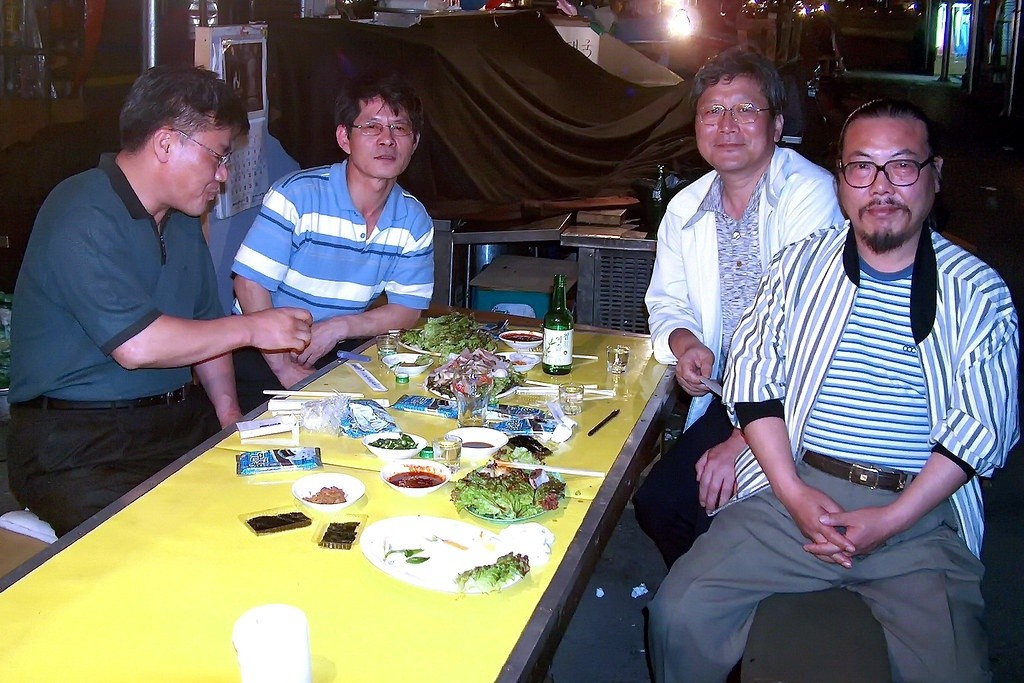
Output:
[606, 345, 630, 374]
[234, 599, 311, 683]
[432, 435, 462, 474]
[559, 383, 584, 416]
[375, 334, 399, 361]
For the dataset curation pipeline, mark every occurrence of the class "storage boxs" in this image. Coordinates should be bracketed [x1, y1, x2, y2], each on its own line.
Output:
[471, 285, 551, 319]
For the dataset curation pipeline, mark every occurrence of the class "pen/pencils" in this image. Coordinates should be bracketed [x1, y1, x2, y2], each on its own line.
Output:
[587, 409, 620, 437]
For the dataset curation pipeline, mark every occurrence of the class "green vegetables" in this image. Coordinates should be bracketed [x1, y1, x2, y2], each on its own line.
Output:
[382, 548, 531, 599]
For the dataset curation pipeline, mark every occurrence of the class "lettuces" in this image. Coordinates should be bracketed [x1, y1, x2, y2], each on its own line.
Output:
[398, 312, 529, 400]
[450, 446, 567, 521]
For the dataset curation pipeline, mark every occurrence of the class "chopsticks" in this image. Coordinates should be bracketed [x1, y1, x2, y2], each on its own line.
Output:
[515, 380, 617, 396]
[518, 351, 599, 360]
[495, 460, 605, 478]
[263, 390, 365, 397]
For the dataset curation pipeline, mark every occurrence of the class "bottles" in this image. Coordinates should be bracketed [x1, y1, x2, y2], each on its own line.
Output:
[650, 164, 669, 229]
[542, 274, 574, 375]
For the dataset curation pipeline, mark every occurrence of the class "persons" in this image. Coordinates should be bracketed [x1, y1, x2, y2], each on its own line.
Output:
[645, 100, 1019, 683]
[630, 44, 848, 682]
[231, 70, 435, 417]
[5, 64, 313, 538]
[21, 28, 92, 102]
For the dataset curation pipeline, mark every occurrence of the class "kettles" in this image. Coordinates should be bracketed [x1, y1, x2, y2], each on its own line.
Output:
[457, 383, 488, 427]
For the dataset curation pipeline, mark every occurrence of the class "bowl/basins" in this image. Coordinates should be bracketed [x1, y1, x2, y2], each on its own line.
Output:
[444, 426, 509, 461]
[491, 352, 542, 373]
[362, 432, 428, 460]
[381, 353, 434, 377]
[291, 473, 365, 512]
[381, 459, 452, 497]
[498, 330, 544, 350]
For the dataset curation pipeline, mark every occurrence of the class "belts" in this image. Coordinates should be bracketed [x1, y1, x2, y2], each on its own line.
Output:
[337, 340, 349, 347]
[798, 446, 916, 493]
[31, 382, 193, 410]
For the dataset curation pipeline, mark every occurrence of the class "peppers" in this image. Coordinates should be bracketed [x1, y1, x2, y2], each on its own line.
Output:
[370, 431, 418, 451]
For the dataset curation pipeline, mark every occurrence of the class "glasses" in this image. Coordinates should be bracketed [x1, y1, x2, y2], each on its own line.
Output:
[840, 156, 937, 188]
[695, 103, 771, 124]
[352, 122, 417, 137]
[169, 127, 233, 166]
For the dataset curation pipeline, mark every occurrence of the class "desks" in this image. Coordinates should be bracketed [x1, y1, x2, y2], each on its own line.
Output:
[1, 306, 679, 683]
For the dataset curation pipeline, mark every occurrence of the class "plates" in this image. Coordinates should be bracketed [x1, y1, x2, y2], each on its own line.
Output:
[395, 328, 498, 358]
[360, 515, 524, 594]
[424, 376, 521, 405]
[458, 465, 567, 522]
